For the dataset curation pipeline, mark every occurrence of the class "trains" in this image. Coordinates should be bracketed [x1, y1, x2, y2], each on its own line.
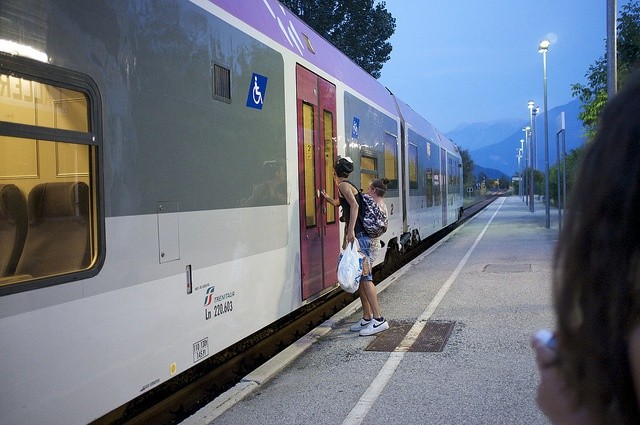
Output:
[0, 0, 462, 425]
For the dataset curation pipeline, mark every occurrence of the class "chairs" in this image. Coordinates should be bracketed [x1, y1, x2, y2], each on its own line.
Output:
[0, 184, 27, 280]
[0, 181, 93, 290]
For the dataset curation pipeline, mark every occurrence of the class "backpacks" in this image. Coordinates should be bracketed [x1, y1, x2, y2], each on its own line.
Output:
[339, 180, 387, 238]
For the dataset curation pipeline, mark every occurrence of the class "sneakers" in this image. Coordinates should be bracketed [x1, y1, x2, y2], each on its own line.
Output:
[350, 318, 370, 331]
[359, 317, 389, 335]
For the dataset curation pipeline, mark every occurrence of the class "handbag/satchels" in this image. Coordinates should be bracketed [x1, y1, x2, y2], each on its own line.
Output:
[336, 241, 362, 293]
[354, 237, 368, 276]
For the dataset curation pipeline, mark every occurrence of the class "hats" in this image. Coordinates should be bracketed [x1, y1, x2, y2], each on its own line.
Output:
[335, 156, 353, 175]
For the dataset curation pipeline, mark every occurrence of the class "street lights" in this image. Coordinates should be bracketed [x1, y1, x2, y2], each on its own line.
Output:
[520, 140, 524, 201]
[519, 149, 522, 200]
[522, 127, 530, 206]
[528, 100, 534, 211]
[538, 40, 550, 229]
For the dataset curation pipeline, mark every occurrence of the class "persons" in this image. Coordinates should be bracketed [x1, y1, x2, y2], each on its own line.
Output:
[321, 156, 391, 337]
